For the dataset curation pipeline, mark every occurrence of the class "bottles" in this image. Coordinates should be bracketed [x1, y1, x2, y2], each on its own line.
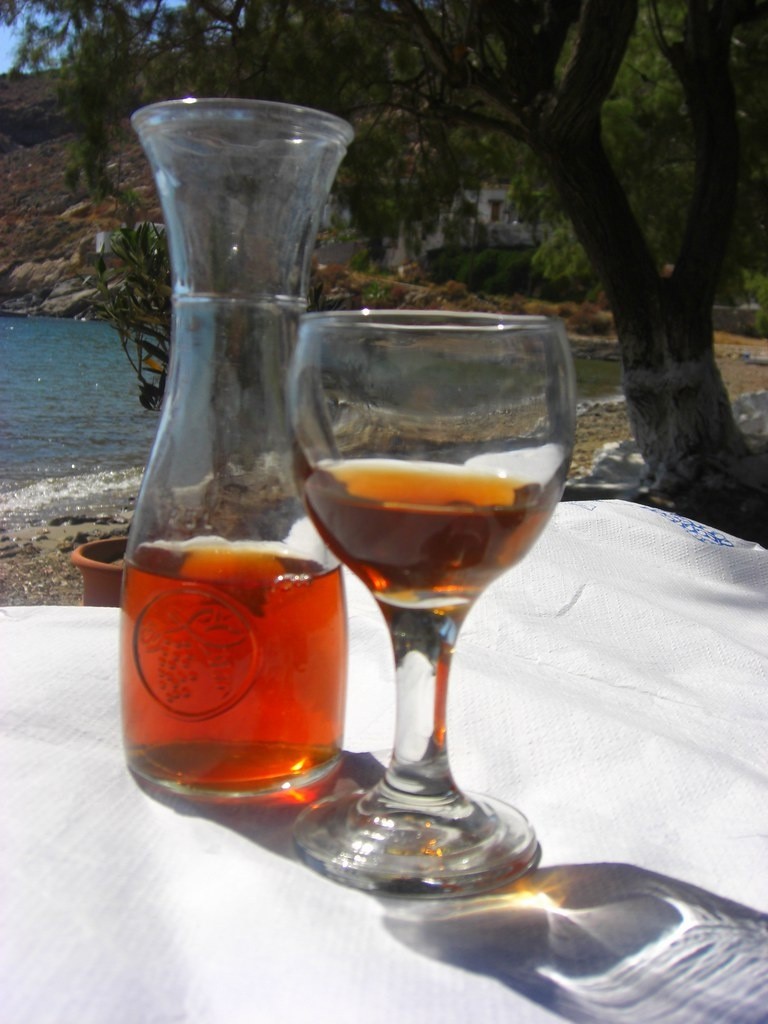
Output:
[120, 96, 358, 824]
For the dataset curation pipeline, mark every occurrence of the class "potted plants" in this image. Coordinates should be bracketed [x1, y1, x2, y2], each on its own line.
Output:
[68, 221, 369, 608]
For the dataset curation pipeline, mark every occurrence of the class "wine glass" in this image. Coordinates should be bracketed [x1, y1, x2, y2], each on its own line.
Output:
[287, 307, 578, 898]
